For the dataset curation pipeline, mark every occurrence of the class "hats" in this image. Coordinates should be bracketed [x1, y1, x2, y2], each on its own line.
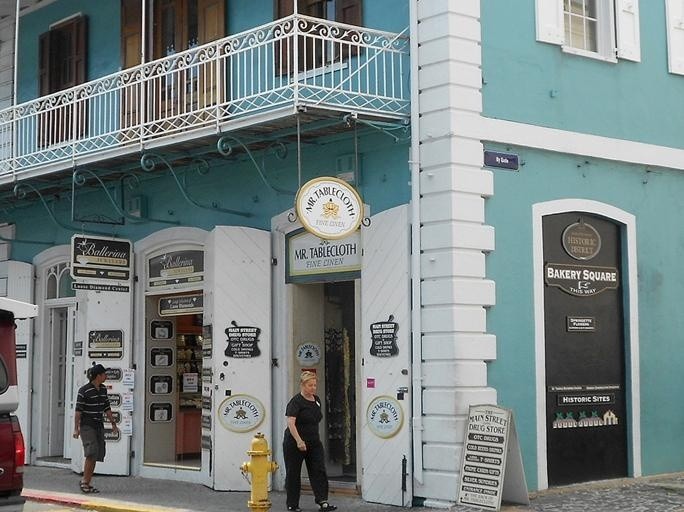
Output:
[88, 364, 111, 379]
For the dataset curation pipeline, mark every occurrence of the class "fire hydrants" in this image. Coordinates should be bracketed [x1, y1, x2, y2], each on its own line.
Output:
[240, 432, 279, 512]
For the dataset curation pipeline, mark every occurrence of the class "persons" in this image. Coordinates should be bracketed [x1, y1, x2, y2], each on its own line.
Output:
[70, 364, 121, 492]
[282, 370, 337, 512]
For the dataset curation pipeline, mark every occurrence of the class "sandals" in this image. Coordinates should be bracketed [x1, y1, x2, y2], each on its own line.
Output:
[317, 501, 336, 511]
[287, 504, 302, 512]
[80, 481, 90, 493]
[89, 487, 100, 493]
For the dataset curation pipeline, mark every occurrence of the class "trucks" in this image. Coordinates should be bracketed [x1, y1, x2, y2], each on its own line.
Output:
[1, 294, 39, 511]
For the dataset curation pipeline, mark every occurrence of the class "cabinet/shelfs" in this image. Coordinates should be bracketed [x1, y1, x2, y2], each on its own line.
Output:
[176, 327, 203, 460]
